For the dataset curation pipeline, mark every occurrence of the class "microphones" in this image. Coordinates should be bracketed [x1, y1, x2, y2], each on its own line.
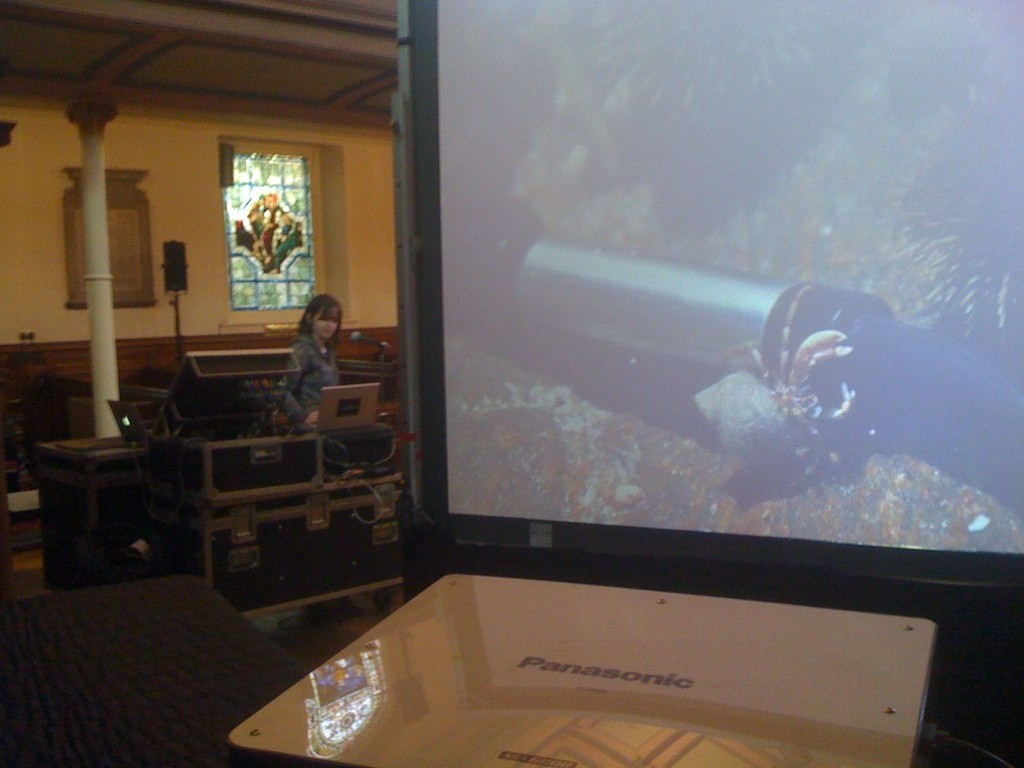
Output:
[350, 330, 391, 349]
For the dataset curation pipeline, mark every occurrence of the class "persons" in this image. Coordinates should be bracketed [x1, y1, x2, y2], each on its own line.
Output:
[275, 295, 342, 469]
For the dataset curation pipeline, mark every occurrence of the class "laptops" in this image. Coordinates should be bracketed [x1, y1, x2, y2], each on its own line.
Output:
[315, 384, 377, 434]
[108, 399, 150, 442]
[226, 572, 938, 768]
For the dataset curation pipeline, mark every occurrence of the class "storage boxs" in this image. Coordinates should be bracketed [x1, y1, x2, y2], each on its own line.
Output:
[154, 347, 301, 442]
[34, 427, 405, 617]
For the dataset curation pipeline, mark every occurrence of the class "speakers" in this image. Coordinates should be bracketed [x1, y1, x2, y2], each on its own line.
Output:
[162, 238, 187, 294]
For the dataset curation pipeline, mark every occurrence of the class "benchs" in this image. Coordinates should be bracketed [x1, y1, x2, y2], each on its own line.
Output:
[42, 354, 398, 439]
